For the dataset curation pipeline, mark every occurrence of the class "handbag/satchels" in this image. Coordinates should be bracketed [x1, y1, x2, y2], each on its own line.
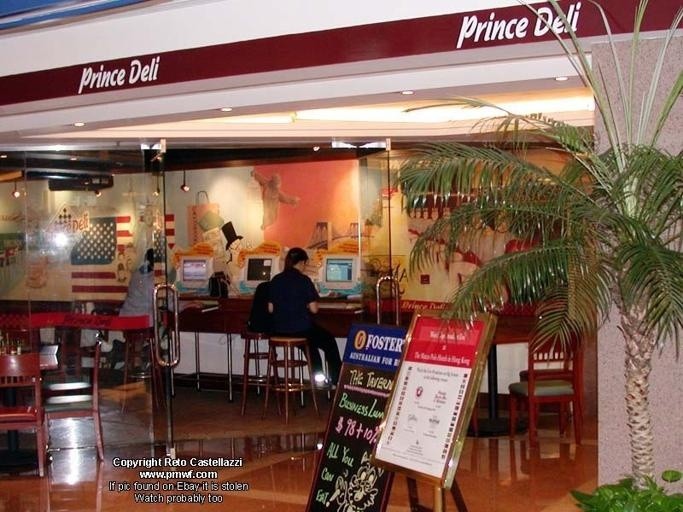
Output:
[209, 271, 228, 298]
[248, 281, 272, 333]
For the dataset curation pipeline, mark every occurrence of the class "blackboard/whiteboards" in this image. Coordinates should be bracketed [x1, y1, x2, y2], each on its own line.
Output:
[304, 361, 395, 512]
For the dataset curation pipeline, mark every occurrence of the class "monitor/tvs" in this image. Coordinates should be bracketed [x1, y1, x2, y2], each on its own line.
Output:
[181, 256, 213, 288]
[322, 253, 361, 290]
[244, 255, 276, 288]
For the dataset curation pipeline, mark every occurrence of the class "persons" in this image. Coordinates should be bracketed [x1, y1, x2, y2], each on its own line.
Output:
[268, 248, 342, 386]
[119, 248, 203, 372]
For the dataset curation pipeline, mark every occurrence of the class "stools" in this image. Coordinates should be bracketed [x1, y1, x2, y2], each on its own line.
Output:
[520, 369, 574, 422]
[118, 330, 164, 414]
[238, 331, 319, 423]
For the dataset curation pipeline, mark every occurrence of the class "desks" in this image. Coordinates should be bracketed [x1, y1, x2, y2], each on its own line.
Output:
[469, 330, 528, 438]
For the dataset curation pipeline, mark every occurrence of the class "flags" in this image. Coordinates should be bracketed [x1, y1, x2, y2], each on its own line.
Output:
[71, 214, 175, 304]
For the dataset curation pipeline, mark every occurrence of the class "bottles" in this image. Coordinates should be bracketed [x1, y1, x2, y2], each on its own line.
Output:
[0, 338, 22, 356]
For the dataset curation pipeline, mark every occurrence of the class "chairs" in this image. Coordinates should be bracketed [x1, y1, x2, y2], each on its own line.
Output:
[0, 329, 104, 478]
[509, 329, 572, 438]
